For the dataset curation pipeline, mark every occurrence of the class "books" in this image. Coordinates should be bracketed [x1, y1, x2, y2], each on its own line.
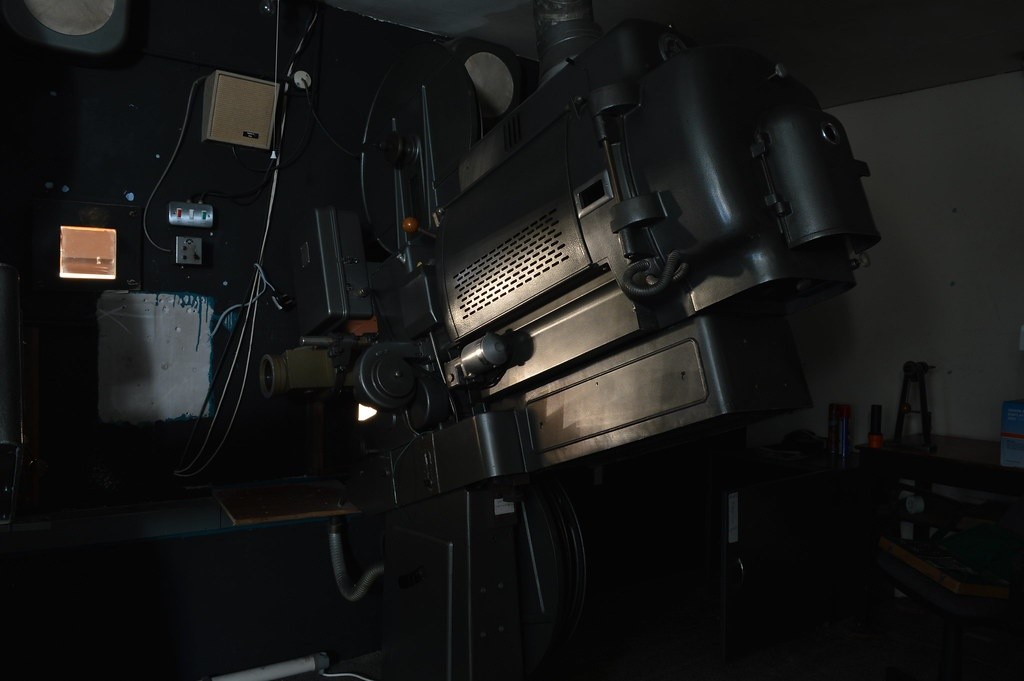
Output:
[878, 536, 1010, 599]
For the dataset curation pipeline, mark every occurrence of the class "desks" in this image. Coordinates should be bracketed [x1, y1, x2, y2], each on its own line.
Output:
[852, 433, 1024, 633]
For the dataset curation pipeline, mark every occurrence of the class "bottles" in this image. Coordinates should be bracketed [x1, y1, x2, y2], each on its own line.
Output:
[839, 405, 849, 456]
[827, 404, 838, 454]
